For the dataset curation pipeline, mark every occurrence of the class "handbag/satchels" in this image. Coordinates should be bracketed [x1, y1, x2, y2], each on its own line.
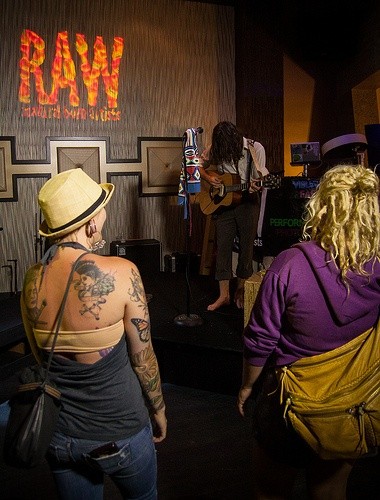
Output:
[267, 316, 380, 461]
[1, 382, 62, 469]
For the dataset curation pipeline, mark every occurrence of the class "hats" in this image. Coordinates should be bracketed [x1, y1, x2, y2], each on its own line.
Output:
[38, 167, 115, 240]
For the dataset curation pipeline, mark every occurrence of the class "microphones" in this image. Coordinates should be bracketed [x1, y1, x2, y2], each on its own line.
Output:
[195, 127, 203, 134]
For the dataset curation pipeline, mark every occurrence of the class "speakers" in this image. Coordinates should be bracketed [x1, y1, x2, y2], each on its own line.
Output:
[110, 238, 161, 277]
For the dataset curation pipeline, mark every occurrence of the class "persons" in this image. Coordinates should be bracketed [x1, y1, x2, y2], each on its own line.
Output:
[19, 167, 167, 500]
[235, 162, 380, 500]
[198, 120, 270, 311]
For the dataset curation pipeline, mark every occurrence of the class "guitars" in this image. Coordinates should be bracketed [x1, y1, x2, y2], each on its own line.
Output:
[198, 170, 281, 216]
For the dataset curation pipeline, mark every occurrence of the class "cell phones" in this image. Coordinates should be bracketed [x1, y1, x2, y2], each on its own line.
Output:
[88, 443, 119, 459]
